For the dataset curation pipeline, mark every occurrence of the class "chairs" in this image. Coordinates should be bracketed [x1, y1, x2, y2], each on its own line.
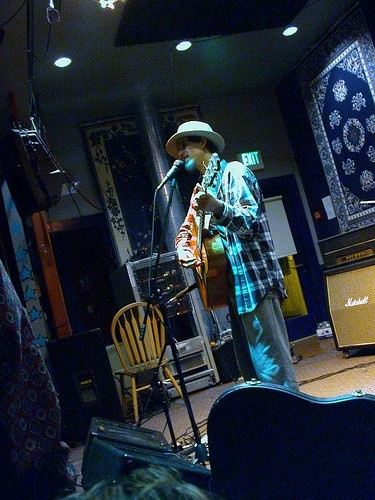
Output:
[113, 302, 183, 423]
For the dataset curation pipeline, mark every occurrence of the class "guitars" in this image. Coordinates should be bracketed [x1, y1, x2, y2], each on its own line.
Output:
[189, 153, 227, 312]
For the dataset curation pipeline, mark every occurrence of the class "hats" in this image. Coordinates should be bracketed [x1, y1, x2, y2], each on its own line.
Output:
[165, 121, 225, 158]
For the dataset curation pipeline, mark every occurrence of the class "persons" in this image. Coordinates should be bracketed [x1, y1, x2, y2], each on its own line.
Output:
[166, 120, 301, 392]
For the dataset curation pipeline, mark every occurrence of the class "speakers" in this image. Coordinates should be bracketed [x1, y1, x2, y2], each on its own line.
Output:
[44, 328, 127, 448]
[324, 258, 375, 350]
[82, 417, 174, 459]
[0, 124, 68, 217]
[81, 437, 212, 495]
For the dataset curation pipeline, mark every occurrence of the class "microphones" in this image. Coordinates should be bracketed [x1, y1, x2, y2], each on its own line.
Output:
[157, 159, 184, 190]
